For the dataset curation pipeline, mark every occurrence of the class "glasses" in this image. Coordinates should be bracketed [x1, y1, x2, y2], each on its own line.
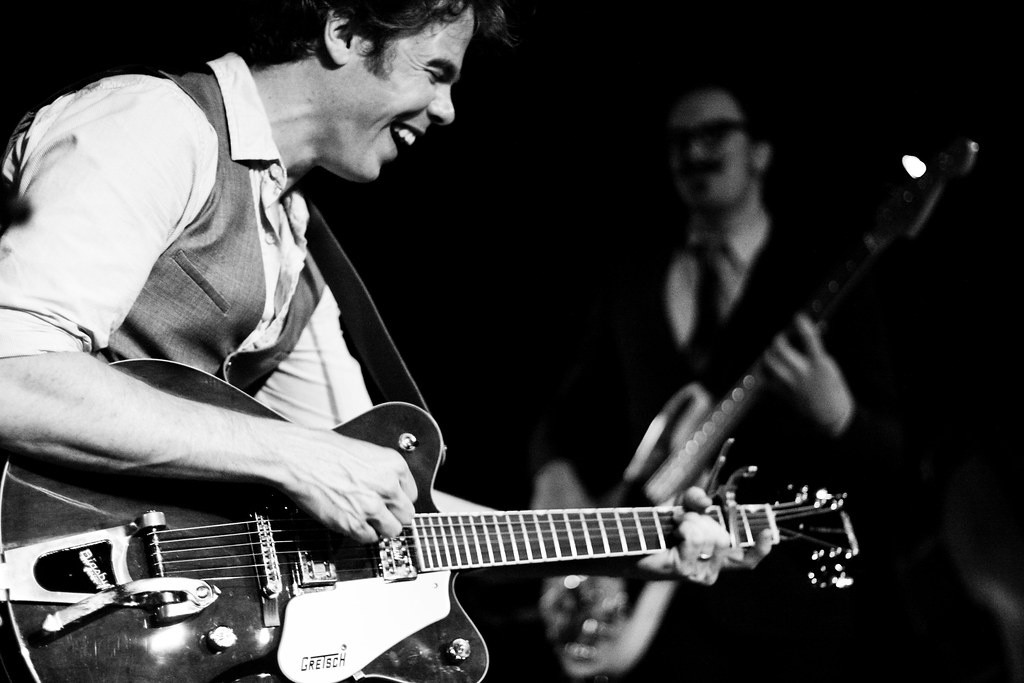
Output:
[664, 121, 749, 147]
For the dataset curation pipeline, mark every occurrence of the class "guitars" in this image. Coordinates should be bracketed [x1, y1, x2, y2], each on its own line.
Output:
[1, 357, 864, 683]
[524, 134, 979, 683]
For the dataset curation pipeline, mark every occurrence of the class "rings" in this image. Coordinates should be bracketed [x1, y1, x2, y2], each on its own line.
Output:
[699, 553, 713, 560]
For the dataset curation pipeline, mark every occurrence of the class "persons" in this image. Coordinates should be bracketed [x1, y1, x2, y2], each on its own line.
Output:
[530, 78, 901, 683]
[0, 2, 771, 683]
